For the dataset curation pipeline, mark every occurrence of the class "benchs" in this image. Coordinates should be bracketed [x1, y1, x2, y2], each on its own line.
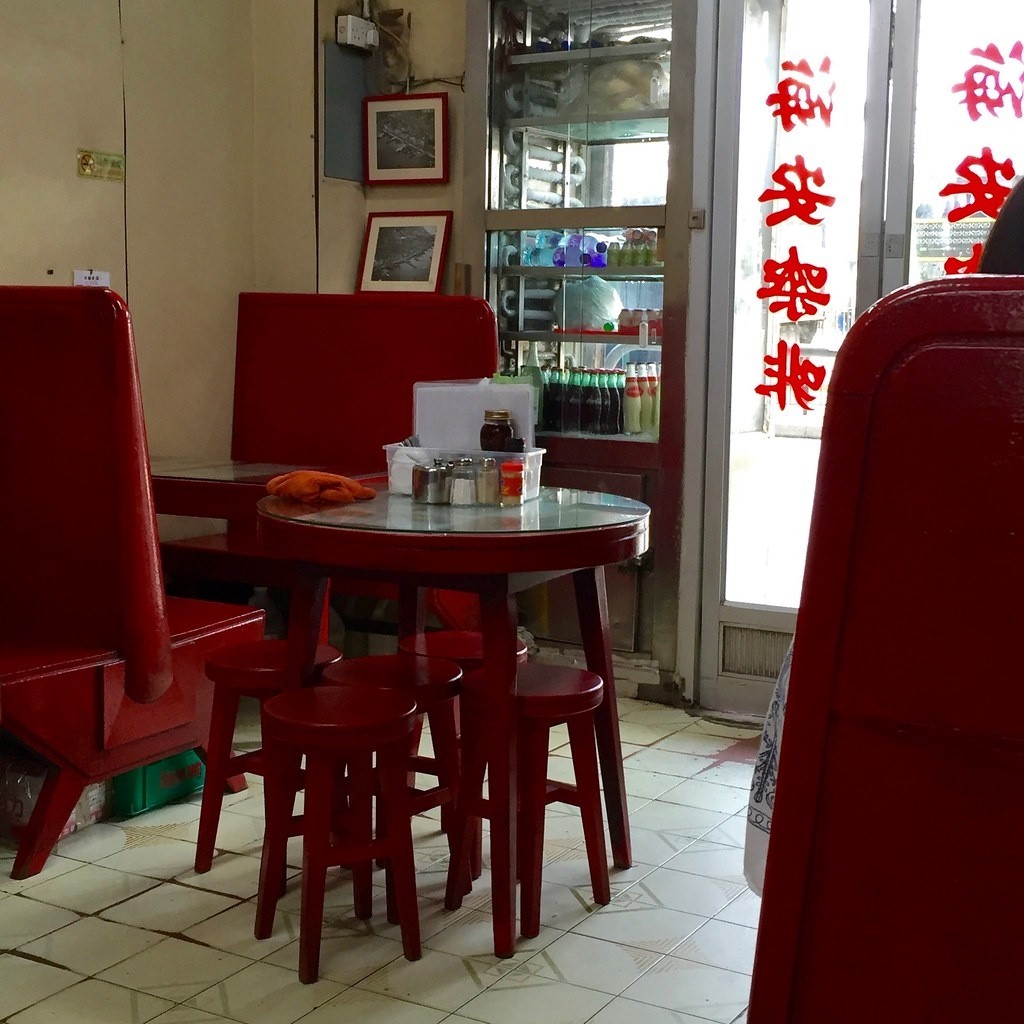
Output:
[230, 292, 499, 632]
[0, 285, 267, 880]
[737, 278, 1024, 1023]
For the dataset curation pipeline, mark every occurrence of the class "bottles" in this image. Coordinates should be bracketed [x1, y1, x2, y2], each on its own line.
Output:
[541, 365, 629, 436]
[449, 458, 501, 506]
[520, 341, 545, 435]
[623, 360, 662, 436]
[607, 242, 663, 268]
[618, 308, 663, 336]
[522, 230, 607, 268]
[622, 227, 657, 244]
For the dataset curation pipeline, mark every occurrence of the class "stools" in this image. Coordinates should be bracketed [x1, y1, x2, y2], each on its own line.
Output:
[194, 632, 612, 985]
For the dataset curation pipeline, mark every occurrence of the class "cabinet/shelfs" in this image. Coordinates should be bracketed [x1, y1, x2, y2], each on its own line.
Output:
[479, 0, 683, 654]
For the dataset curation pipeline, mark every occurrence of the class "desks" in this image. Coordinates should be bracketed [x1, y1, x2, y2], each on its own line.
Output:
[255, 483, 651, 959]
[150, 459, 393, 522]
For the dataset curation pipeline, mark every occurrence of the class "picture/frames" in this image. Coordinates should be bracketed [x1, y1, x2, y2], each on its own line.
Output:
[355, 212, 455, 293]
[363, 93, 450, 185]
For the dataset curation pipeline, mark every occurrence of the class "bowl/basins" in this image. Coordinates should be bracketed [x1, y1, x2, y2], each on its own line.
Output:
[390, 447, 426, 490]
